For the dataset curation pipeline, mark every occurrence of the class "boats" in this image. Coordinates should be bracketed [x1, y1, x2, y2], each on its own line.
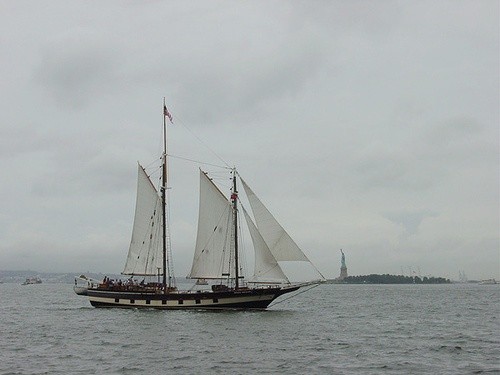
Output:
[21, 276, 41, 285]
[74, 274, 102, 289]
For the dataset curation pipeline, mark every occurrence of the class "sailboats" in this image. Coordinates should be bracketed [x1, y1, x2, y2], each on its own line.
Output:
[72, 94, 327, 311]
